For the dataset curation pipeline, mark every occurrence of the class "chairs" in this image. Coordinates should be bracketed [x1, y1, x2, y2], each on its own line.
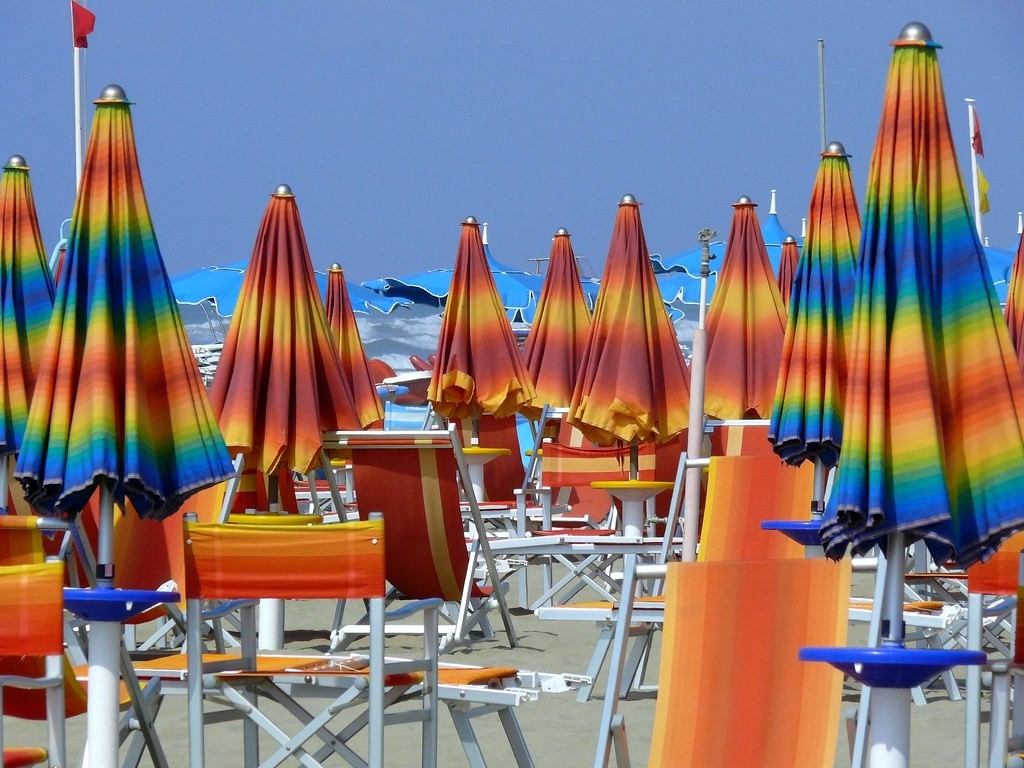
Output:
[0, 400, 1024, 768]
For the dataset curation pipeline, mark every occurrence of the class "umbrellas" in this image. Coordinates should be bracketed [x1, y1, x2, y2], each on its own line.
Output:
[426, 216, 537, 449]
[13, 86, 236, 593]
[568, 194, 691, 482]
[0, 20, 1024, 679]
[208, 183, 363, 512]
